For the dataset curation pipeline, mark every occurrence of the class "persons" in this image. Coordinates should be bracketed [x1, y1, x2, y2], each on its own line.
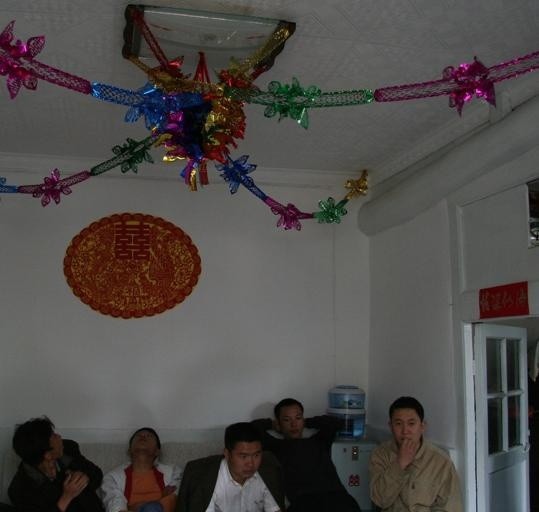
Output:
[368, 395, 463, 512]
[173, 421, 288, 512]
[6, 415, 107, 511]
[99, 427, 184, 512]
[249, 397, 363, 512]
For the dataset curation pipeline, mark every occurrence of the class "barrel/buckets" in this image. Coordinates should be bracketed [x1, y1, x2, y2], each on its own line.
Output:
[327, 384, 366, 443]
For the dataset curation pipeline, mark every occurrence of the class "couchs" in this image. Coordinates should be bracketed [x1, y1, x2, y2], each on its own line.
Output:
[0, 442, 224, 504]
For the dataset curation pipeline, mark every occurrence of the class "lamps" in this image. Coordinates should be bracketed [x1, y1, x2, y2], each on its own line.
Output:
[121, 4, 296, 72]
